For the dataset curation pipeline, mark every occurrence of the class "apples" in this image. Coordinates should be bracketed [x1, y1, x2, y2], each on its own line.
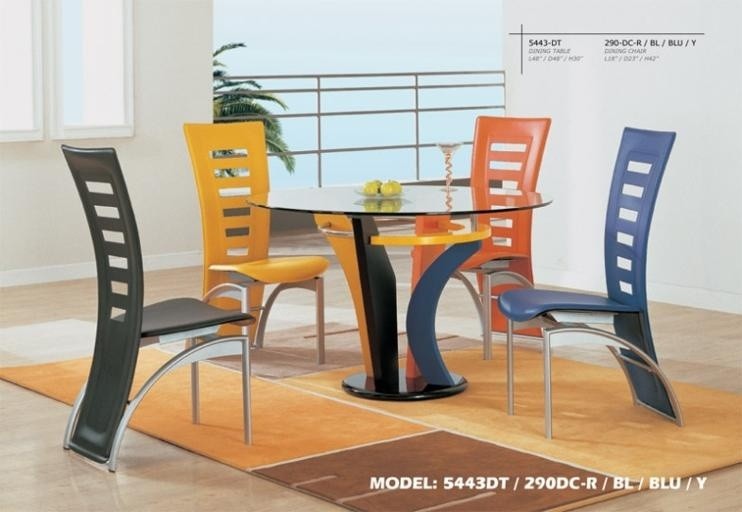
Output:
[364, 199, 401, 213]
[364, 179, 401, 196]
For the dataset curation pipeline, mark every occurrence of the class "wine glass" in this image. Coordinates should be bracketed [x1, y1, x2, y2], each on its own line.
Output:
[436, 140, 463, 193]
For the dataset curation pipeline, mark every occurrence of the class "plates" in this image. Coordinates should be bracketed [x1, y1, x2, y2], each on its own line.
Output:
[348, 185, 412, 199]
